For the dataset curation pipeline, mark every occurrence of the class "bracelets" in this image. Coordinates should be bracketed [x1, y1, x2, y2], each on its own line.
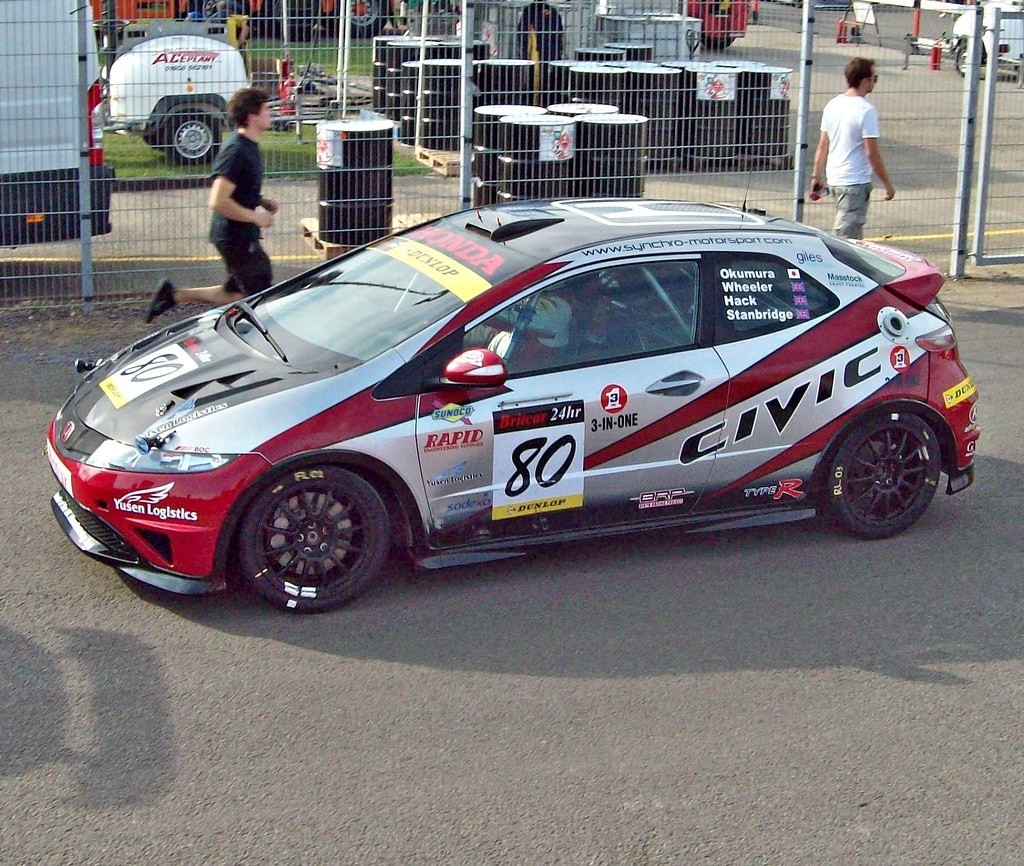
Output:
[811, 174, 818, 178]
[259, 195, 265, 203]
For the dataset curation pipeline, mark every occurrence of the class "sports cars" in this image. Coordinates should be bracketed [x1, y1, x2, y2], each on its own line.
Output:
[45, 197, 979, 617]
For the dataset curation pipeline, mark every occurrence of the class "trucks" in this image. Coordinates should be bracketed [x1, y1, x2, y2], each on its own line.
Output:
[678, 0, 748, 52]
[103, 1, 391, 165]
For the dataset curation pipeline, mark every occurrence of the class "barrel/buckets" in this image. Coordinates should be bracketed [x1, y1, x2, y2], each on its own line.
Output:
[317, 36, 792, 245]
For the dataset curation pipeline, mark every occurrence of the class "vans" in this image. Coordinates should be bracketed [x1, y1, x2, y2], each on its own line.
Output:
[0, 0, 116, 245]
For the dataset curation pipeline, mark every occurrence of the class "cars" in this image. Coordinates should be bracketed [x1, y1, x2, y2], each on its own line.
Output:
[952, 0, 1024, 79]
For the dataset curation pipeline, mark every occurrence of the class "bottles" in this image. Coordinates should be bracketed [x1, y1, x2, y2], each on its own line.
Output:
[810, 187, 830, 201]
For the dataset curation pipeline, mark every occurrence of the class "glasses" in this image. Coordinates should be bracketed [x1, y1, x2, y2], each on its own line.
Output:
[866, 75, 878, 83]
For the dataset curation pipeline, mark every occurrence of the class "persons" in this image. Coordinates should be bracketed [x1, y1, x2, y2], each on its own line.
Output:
[389, 0, 399, 28]
[206, 0, 249, 76]
[146, 90, 279, 326]
[482, 278, 581, 373]
[809, 56, 895, 238]
[938, 0, 960, 21]
[186, 0, 203, 22]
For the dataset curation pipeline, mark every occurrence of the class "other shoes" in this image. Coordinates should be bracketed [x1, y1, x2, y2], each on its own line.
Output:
[145, 277, 175, 324]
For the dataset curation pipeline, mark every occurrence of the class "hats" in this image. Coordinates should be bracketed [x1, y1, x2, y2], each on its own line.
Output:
[188, 11, 203, 22]
